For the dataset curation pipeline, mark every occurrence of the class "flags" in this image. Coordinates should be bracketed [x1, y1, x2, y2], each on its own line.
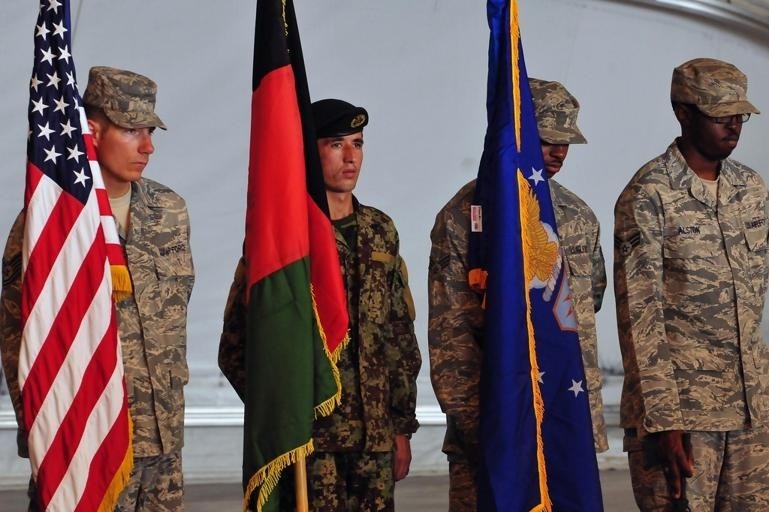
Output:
[243, 0, 350, 512]
[467, 0, 603, 512]
[18, 0, 134, 511]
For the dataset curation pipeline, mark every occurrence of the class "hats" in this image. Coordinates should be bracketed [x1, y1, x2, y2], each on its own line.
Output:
[84, 66, 166, 130]
[671, 58, 760, 117]
[527, 77, 588, 145]
[312, 99, 368, 137]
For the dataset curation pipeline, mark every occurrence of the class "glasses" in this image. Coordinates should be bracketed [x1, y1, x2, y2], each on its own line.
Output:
[716, 113, 750, 124]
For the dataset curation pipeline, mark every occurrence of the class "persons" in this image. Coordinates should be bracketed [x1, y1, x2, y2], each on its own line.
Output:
[428, 76, 608, 512]
[2, 65, 195, 512]
[218, 99, 421, 511]
[614, 58, 768, 512]
